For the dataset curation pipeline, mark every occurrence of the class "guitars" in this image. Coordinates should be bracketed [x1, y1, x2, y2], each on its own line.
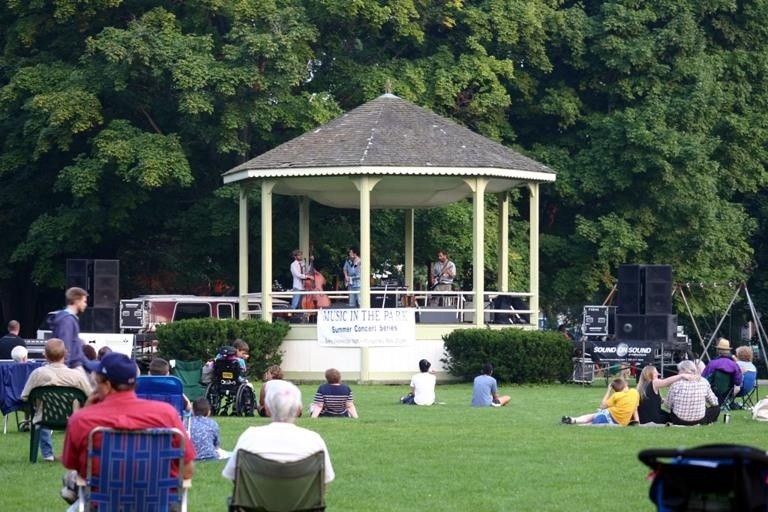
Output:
[343, 273, 359, 284]
[427, 265, 453, 289]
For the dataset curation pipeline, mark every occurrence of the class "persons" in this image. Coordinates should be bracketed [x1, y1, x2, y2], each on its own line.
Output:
[562, 378, 640, 427]
[185, 397, 220, 459]
[222, 380, 335, 512]
[692, 358, 705, 376]
[343, 248, 361, 308]
[201, 339, 249, 386]
[664, 360, 720, 427]
[98, 346, 111, 362]
[1, 320, 27, 360]
[310, 368, 359, 419]
[733, 346, 757, 396]
[81, 345, 96, 361]
[431, 251, 456, 307]
[287, 250, 311, 318]
[637, 365, 693, 424]
[471, 365, 510, 407]
[701, 338, 742, 410]
[60, 353, 197, 512]
[52, 287, 89, 371]
[256, 365, 304, 418]
[21, 339, 94, 461]
[401, 360, 437, 406]
[147, 359, 191, 427]
[11, 345, 37, 432]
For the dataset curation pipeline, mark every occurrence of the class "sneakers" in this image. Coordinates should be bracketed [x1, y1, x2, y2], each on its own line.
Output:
[561, 416, 576, 425]
[60, 470, 79, 504]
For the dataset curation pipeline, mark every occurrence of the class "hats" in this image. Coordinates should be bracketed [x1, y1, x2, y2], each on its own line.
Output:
[85, 352, 137, 384]
[715, 337, 732, 349]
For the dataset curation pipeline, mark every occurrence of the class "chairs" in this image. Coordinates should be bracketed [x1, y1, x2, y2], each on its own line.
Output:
[26, 383, 88, 465]
[708, 370, 736, 415]
[732, 369, 760, 410]
[0, 363, 40, 434]
[77, 425, 188, 512]
[134, 376, 183, 423]
[226, 447, 327, 512]
[170, 357, 205, 402]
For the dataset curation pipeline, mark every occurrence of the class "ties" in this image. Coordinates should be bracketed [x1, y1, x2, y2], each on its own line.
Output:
[299, 262, 303, 285]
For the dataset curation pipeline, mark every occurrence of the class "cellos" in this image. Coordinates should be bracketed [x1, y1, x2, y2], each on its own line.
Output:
[300, 240, 332, 309]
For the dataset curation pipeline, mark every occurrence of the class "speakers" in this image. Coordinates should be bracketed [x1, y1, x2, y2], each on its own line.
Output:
[615, 313, 644, 339]
[617, 263, 644, 313]
[94, 259, 119, 306]
[642, 265, 673, 313]
[645, 316, 678, 341]
[64, 259, 92, 308]
[92, 309, 119, 332]
